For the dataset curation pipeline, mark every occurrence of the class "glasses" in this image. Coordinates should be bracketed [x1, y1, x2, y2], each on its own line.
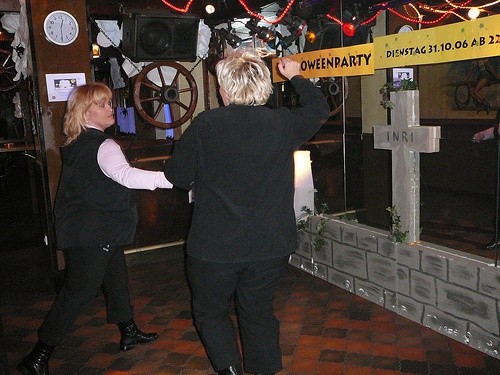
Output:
[98, 100, 113, 109]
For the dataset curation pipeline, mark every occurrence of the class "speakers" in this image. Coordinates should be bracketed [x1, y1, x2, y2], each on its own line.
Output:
[123, 8, 200, 62]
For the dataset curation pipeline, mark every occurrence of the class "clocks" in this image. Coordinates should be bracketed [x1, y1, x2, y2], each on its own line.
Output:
[43, 10, 79, 46]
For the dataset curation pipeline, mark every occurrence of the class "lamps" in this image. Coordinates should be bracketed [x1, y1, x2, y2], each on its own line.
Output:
[275, 9, 301, 33]
[244, 17, 275, 42]
[218, 27, 241, 49]
[198, 0, 231, 27]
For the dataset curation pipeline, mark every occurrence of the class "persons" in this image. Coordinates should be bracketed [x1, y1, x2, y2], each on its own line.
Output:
[13, 82, 174, 375]
[485, 238, 500, 250]
[162, 48, 331, 375]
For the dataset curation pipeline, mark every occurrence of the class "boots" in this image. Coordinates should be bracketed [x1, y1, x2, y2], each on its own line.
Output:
[16, 340, 54, 375]
[118, 321, 157, 352]
